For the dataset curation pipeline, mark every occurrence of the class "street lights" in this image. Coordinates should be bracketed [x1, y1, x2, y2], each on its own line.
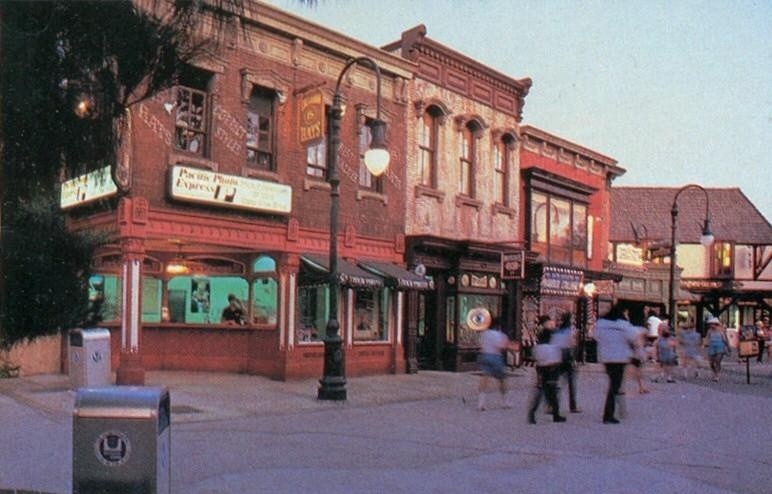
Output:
[313, 56, 392, 400]
[667, 182, 715, 340]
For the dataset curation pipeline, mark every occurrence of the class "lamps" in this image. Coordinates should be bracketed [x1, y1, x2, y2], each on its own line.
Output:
[166, 239, 190, 274]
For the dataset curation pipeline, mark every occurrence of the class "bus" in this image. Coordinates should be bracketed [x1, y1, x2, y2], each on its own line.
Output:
[676, 299, 772, 349]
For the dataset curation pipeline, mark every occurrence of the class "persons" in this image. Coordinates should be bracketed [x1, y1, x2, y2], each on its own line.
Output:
[619, 309, 772, 396]
[527, 328, 567, 425]
[476, 317, 515, 410]
[592, 305, 640, 424]
[221, 295, 248, 325]
[544, 312, 582, 412]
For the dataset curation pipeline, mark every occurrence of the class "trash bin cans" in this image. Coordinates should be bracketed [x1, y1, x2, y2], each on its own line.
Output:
[68, 327, 111, 396]
[72, 384, 170, 494]
